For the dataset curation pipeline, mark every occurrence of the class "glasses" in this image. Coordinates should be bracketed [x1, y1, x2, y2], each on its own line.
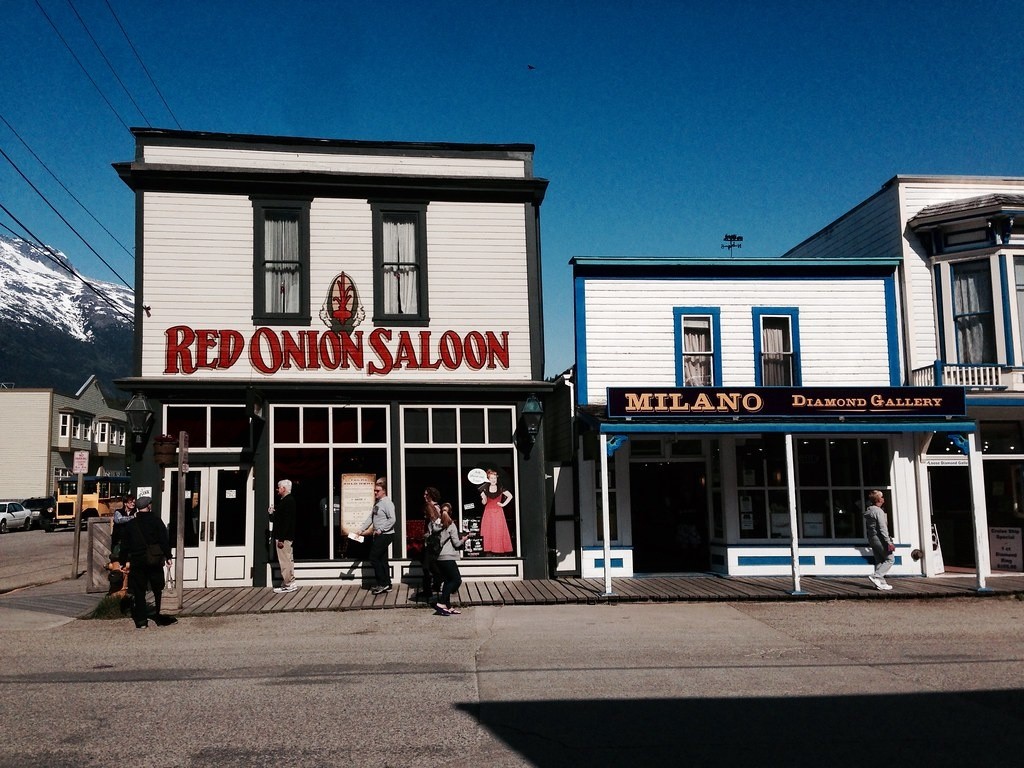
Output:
[374, 489, 382, 493]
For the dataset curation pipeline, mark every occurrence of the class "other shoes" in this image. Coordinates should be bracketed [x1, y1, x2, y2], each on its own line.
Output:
[273, 583, 298, 593]
[416, 590, 434, 597]
[372, 585, 389, 595]
[370, 584, 393, 591]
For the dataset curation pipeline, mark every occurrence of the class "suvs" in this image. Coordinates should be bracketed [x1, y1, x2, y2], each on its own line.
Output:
[20, 496, 56, 532]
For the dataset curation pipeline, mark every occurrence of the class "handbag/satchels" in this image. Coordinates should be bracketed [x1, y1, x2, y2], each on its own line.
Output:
[160, 566, 180, 615]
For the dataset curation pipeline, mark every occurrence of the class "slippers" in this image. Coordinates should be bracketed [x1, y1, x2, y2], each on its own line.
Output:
[448, 607, 461, 614]
[434, 601, 451, 618]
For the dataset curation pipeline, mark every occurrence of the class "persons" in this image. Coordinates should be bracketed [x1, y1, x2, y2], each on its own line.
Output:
[480, 470, 513, 553]
[112, 494, 178, 628]
[357, 482, 470, 616]
[269, 480, 298, 593]
[866, 490, 896, 589]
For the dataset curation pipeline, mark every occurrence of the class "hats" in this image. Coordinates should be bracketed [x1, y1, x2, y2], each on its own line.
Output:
[136, 497, 152, 510]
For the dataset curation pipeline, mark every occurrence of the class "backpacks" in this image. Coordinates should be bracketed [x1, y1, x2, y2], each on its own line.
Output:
[424, 520, 452, 563]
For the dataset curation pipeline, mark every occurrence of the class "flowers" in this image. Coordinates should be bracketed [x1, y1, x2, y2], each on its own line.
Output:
[154, 434, 179, 443]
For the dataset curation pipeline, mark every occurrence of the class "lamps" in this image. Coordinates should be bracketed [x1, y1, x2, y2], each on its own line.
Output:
[125, 390, 154, 444]
[522, 393, 543, 443]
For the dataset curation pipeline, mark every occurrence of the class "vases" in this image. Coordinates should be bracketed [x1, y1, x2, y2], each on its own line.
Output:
[154, 442, 174, 464]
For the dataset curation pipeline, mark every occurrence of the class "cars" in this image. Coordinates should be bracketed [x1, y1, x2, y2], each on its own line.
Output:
[0, 502, 32, 534]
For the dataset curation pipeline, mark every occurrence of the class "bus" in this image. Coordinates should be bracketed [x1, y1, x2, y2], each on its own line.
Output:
[56, 475, 132, 531]
